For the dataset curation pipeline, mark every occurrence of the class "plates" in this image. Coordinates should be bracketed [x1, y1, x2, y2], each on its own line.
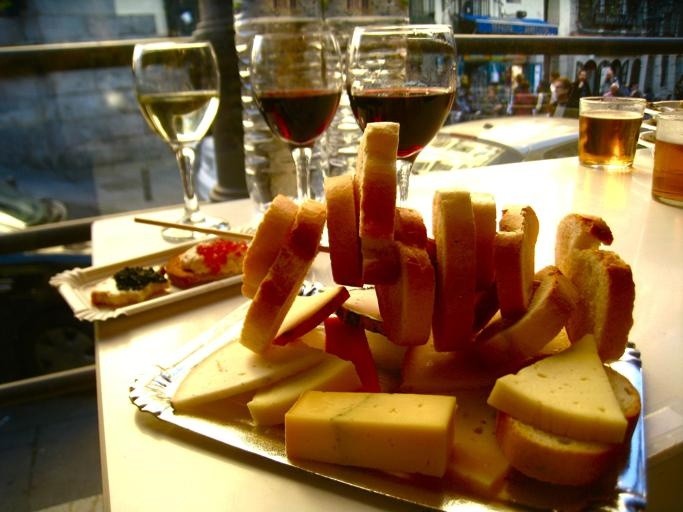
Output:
[46, 237, 245, 323]
[128, 298, 647, 512]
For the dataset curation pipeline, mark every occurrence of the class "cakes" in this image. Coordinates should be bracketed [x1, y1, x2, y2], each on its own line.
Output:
[91, 238, 248, 306]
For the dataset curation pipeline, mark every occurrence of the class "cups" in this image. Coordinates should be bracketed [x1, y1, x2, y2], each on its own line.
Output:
[578, 96, 647, 172]
[651, 110, 683, 208]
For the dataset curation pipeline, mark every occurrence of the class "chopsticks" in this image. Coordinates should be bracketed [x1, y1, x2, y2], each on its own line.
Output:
[134, 217, 330, 253]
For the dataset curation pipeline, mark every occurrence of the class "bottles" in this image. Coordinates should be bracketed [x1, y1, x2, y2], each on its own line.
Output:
[321, 1, 410, 178]
[233, 0, 329, 212]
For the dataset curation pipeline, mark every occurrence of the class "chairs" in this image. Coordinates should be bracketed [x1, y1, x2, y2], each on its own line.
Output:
[511, 88, 579, 120]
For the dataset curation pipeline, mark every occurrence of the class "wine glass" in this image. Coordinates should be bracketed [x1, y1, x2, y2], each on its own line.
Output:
[344, 22, 457, 205]
[131, 43, 232, 241]
[250, 31, 344, 283]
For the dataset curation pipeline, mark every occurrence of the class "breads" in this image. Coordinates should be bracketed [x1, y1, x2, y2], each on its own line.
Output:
[158, 118, 640, 488]
